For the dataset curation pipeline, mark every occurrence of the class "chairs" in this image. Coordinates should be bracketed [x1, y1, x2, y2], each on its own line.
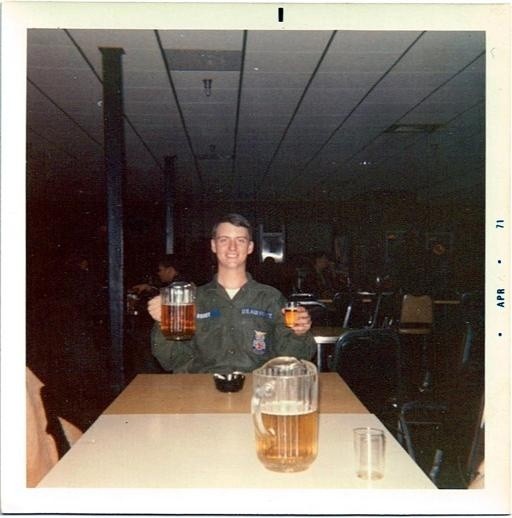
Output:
[35, 292, 478, 488]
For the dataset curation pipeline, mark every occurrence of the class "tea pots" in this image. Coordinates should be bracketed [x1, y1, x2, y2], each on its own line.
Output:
[251, 356, 321, 474]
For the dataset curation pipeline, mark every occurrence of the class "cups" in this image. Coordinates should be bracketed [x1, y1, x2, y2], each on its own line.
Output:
[284, 301, 299, 328]
[352, 427, 386, 481]
[159, 280, 198, 341]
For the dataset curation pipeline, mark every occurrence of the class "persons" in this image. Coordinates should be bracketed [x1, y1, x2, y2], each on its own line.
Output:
[300, 250, 338, 327]
[126, 252, 189, 375]
[147, 213, 317, 376]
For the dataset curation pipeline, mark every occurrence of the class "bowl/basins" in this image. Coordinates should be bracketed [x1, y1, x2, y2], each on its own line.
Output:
[212, 373, 246, 393]
